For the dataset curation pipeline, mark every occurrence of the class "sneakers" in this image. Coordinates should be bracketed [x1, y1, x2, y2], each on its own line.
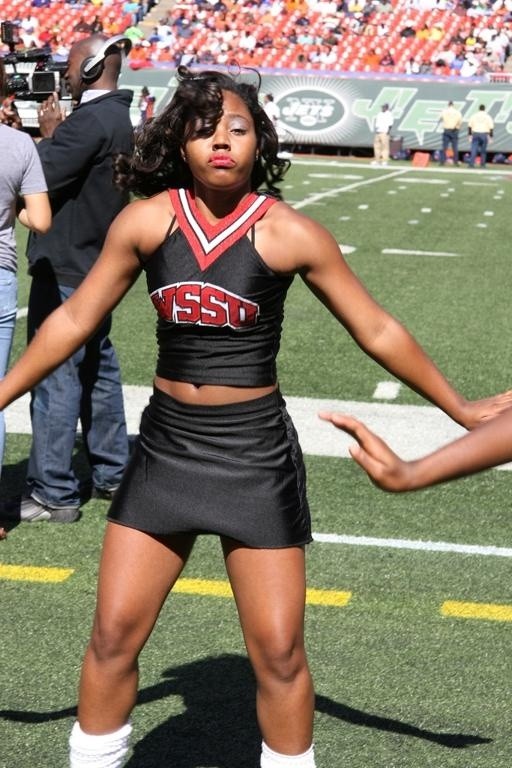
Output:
[0, 496, 79, 522]
[80, 483, 119, 499]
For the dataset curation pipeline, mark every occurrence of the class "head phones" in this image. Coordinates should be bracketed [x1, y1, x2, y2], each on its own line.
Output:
[80, 35, 132, 81]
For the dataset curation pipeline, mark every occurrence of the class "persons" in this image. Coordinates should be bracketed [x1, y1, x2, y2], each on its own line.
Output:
[318, 404, 512, 494]
[1, 0, 512, 79]
[0, 75, 512, 763]
[1, 35, 136, 540]
[367, 103, 492, 170]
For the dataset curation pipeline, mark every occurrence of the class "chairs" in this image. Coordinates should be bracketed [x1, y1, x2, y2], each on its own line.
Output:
[0, 0, 512, 76]
